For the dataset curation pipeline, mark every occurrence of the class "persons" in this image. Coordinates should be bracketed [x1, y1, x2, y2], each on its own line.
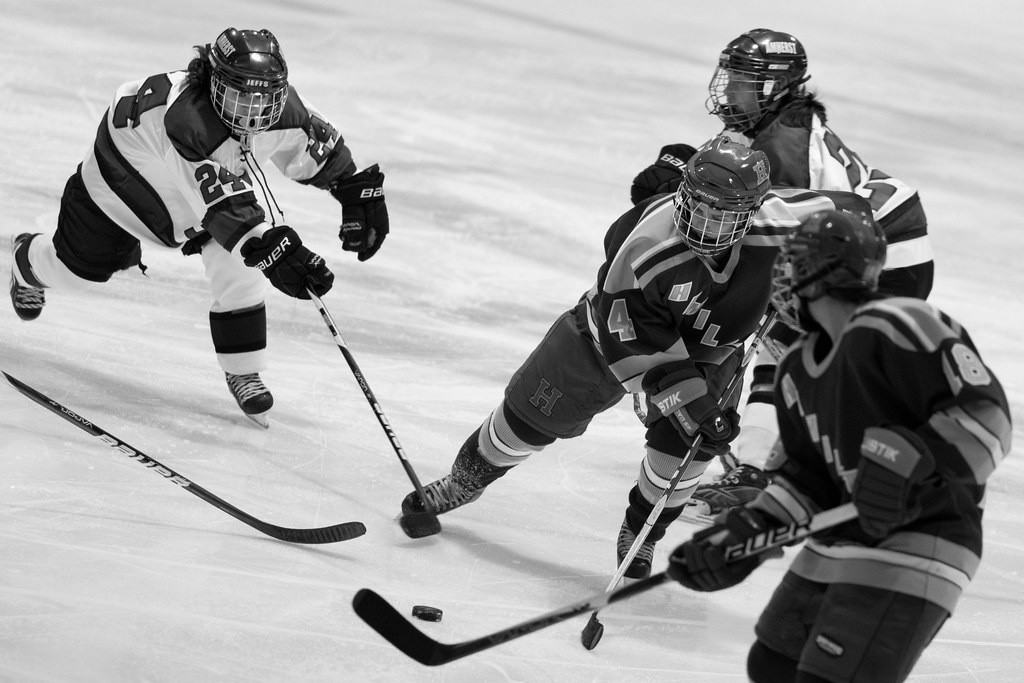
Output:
[9, 27, 390, 430]
[632, 28, 936, 526]
[668, 208, 1013, 683]
[401, 136, 875, 595]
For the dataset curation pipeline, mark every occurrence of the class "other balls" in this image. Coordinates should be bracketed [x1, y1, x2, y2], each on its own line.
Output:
[410, 605, 444, 623]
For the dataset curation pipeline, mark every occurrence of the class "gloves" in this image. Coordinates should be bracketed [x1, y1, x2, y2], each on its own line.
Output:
[240, 224, 335, 301]
[630, 141, 697, 205]
[666, 503, 809, 593]
[850, 419, 937, 541]
[640, 357, 743, 456]
[329, 163, 390, 262]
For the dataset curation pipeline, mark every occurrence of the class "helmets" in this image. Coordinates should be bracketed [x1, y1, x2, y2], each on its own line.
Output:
[668, 136, 773, 257]
[204, 26, 289, 141]
[768, 207, 888, 337]
[705, 28, 808, 136]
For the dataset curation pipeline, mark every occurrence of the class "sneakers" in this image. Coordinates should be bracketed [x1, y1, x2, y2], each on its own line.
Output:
[8, 231, 47, 321]
[677, 450, 771, 528]
[399, 473, 487, 518]
[616, 515, 659, 587]
[224, 370, 275, 429]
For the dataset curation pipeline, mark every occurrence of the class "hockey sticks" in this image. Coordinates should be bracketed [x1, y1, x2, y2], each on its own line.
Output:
[752, 322, 783, 362]
[578, 307, 782, 653]
[349, 502, 860, 667]
[305, 281, 444, 541]
[3, 369, 370, 546]
